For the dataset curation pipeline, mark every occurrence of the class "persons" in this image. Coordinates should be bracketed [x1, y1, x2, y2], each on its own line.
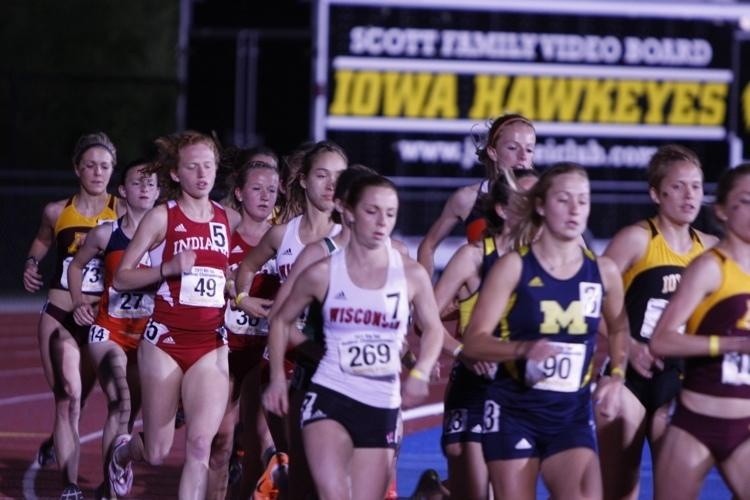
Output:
[219, 147, 286, 224]
[207, 160, 279, 500]
[463, 162, 630, 500]
[252, 166, 411, 499]
[24, 132, 126, 500]
[263, 177, 441, 500]
[67, 156, 160, 500]
[648, 163, 750, 500]
[409, 169, 590, 500]
[418, 114, 536, 280]
[111, 127, 243, 500]
[236, 140, 350, 319]
[598, 146, 720, 500]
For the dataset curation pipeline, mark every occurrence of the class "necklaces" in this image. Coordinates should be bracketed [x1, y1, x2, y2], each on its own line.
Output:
[535, 243, 584, 272]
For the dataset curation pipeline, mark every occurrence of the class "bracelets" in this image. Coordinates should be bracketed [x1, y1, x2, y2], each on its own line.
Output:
[452, 343, 465, 356]
[236, 291, 249, 305]
[160, 261, 166, 278]
[225, 278, 236, 290]
[409, 368, 429, 383]
[709, 335, 720, 356]
[611, 367, 625, 378]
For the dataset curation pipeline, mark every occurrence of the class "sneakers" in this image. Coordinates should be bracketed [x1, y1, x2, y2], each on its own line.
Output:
[228, 451, 244, 487]
[176, 409, 185, 429]
[254, 453, 290, 499]
[410, 469, 439, 500]
[63, 485, 84, 500]
[38, 434, 57, 465]
[110, 433, 133, 496]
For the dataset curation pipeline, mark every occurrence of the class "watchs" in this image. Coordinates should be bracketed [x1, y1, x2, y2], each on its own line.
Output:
[25, 256, 39, 267]
